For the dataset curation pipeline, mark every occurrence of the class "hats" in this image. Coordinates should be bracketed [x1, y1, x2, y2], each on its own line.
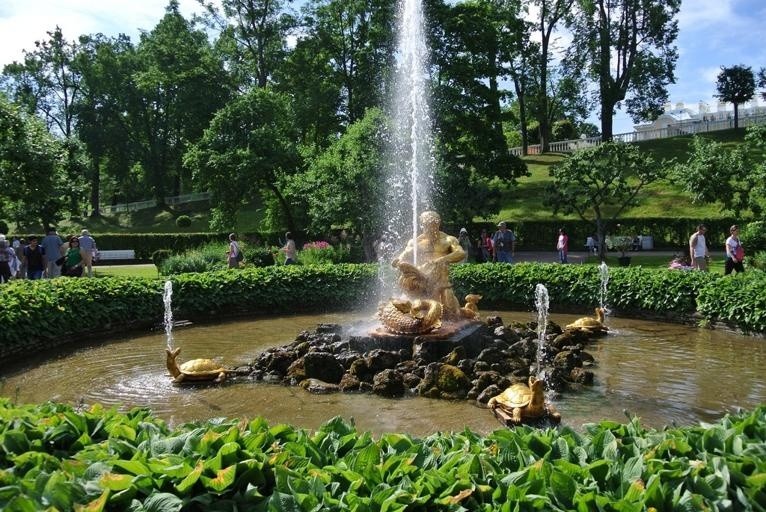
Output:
[497, 221, 506, 227]
[459, 228, 467, 234]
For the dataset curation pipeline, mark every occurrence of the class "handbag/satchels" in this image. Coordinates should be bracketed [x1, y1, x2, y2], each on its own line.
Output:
[55, 256, 65, 266]
[235, 251, 243, 262]
[733, 246, 745, 262]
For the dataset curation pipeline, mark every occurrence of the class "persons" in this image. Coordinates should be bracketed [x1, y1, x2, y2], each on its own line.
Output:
[724, 224, 745, 274]
[393, 210, 466, 322]
[457, 221, 515, 265]
[689, 224, 710, 271]
[282, 232, 296, 265]
[226, 233, 241, 269]
[556, 228, 568, 264]
[0, 220, 100, 284]
